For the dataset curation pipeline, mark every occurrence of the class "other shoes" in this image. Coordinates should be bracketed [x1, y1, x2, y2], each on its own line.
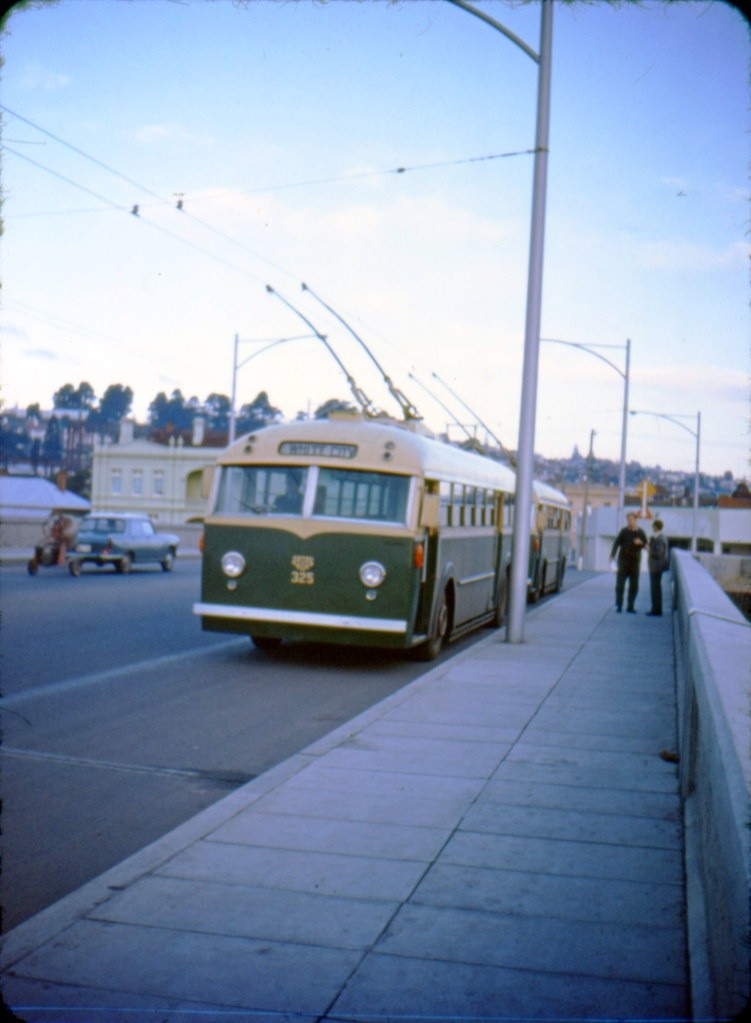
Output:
[616, 604, 622, 613]
[627, 608, 637, 613]
[646, 610, 662, 616]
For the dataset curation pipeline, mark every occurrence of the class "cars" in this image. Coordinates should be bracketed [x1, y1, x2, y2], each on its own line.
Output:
[65, 511, 181, 577]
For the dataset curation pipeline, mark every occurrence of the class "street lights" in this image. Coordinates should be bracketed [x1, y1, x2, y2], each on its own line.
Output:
[630, 410, 703, 554]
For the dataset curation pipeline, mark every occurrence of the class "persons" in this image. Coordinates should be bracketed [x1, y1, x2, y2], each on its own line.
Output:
[274, 470, 304, 515]
[107, 520, 116, 531]
[51, 512, 74, 544]
[646, 520, 670, 617]
[609, 512, 648, 614]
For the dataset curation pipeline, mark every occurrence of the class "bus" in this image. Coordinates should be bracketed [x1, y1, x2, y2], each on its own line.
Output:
[192, 283, 574, 665]
